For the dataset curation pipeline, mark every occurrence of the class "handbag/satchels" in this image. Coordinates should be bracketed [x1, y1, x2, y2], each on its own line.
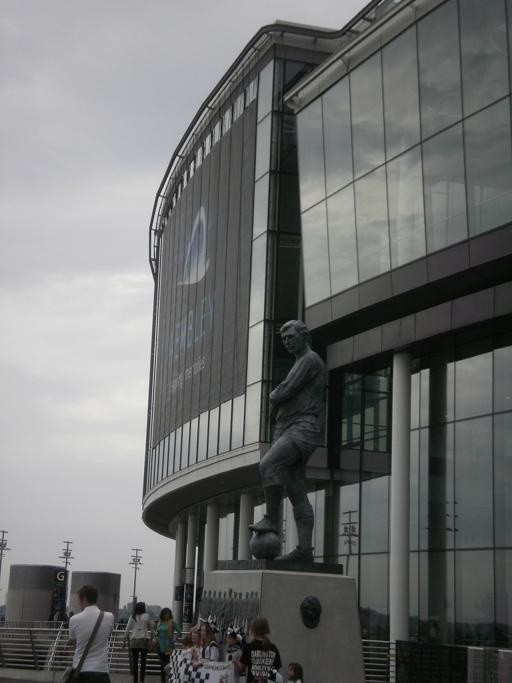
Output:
[60, 666, 81, 683]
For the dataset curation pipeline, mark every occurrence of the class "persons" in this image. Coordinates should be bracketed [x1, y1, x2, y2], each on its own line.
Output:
[69, 584, 116, 682]
[250, 318, 330, 559]
[49, 608, 74, 637]
[120, 602, 304, 682]
